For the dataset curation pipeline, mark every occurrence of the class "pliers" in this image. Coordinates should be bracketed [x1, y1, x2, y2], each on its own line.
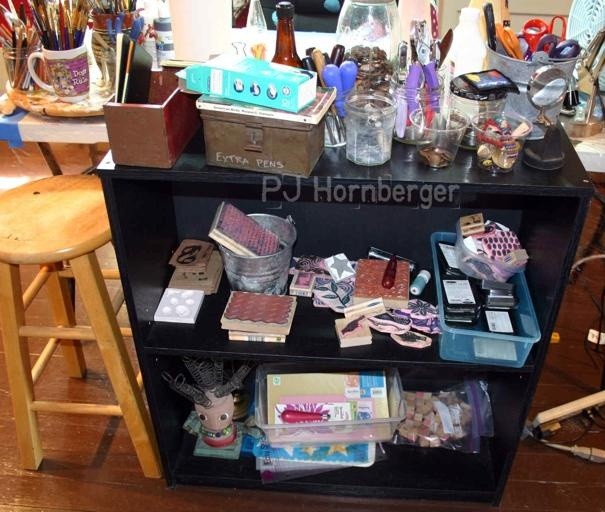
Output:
[405, 22, 439, 115]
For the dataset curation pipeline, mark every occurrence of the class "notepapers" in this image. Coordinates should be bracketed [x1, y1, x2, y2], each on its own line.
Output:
[334, 317, 374, 348]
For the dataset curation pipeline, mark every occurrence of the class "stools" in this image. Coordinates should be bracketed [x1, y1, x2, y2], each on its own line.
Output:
[0, 174, 164, 478]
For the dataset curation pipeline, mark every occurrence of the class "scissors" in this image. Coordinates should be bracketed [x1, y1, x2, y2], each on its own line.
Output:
[482, 3, 579, 61]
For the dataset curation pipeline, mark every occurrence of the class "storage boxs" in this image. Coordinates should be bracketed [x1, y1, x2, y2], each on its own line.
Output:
[201, 107, 325, 179]
[253, 361, 408, 449]
[430, 231, 542, 369]
[102, 66, 202, 169]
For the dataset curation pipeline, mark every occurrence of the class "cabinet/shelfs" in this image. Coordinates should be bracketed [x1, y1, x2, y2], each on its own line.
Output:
[93, 121, 596, 507]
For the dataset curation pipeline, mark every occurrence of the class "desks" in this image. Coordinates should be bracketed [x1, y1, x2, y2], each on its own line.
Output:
[566, 127, 605, 283]
[0, 94, 109, 310]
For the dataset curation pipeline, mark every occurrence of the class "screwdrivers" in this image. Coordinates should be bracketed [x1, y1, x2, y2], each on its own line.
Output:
[301, 44, 358, 151]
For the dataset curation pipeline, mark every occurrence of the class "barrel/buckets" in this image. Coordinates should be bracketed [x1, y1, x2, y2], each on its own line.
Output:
[483, 34, 589, 140]
[218, 212, 298, 297]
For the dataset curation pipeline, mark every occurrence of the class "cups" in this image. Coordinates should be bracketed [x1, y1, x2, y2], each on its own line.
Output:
[450, 76, 506, 151]
[93, 10, 142, 93]
[1, 41, 32, 93]
[314, 85, 358, 147]
[28, 33, 91, 103]
[388, 70, 447, 144]
[409, 109, 469, 169]
[344, 91, 395, 166]
[144, 39, 162, 72]
[468, 111, 534, 176]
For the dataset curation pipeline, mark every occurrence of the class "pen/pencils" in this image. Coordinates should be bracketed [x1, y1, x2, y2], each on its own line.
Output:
[30, 0, 90, 50]
[91, 12, 144, 52]
[89, 0, 137, 15]
[0, 0, 42, 90]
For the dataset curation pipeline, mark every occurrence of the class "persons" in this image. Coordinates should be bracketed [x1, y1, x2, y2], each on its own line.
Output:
[296, 271, 311, 288]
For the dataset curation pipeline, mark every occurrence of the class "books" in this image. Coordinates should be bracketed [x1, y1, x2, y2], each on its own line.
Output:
[195, 80, 339, 127]
[350, 255, 412, 310]
[152, 200, 298, 345]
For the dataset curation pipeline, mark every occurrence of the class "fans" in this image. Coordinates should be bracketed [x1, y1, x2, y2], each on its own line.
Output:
[565, 0, 605, 117]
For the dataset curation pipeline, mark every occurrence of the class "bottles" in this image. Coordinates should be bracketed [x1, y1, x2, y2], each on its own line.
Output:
[333, 0, 400, 89]
[152, 1, 175, 68]
[270, 1, 304, 69]
[411, 268, 431, 296]
